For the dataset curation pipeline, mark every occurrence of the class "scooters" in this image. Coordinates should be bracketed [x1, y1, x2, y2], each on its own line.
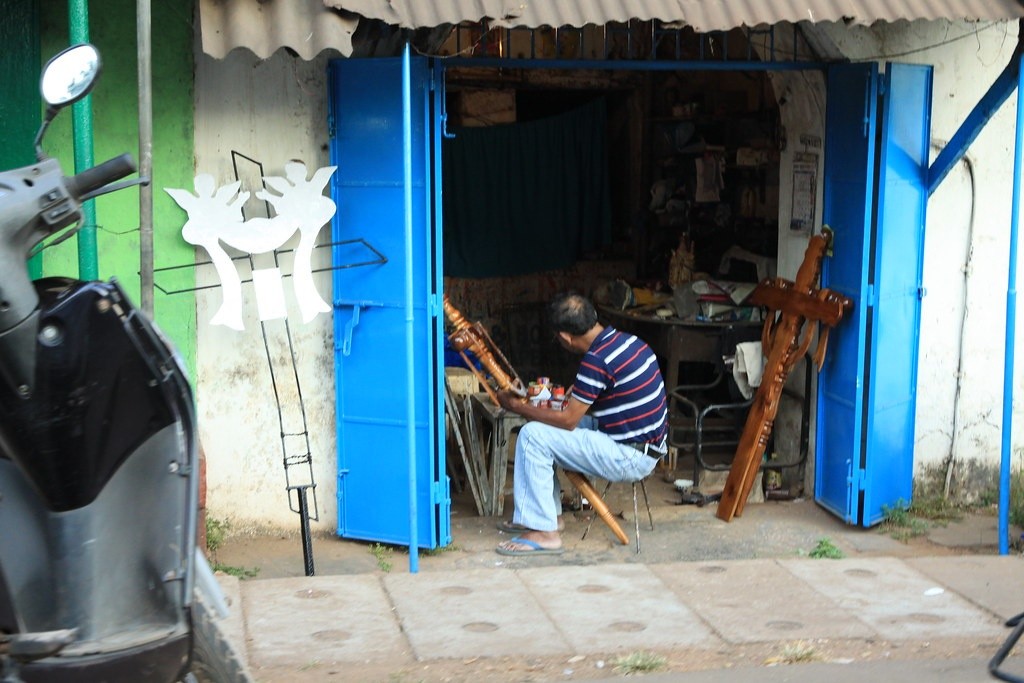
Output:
[0, 44, 257, 683]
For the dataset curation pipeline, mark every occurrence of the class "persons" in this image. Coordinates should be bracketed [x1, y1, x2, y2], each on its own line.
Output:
[497, 295, 669, 556]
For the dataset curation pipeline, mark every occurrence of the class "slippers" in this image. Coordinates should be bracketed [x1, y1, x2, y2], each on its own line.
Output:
[496, 520, 533, 533]
[496, 536, 565, 555]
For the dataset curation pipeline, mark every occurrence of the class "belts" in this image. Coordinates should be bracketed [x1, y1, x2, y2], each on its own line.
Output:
[627, 443, 663, 459]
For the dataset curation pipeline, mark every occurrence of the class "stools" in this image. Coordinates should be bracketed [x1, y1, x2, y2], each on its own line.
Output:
[582, 470, 656, 554]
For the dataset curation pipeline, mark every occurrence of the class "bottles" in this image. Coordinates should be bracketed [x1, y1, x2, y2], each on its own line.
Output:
[765, 453, 782, 490]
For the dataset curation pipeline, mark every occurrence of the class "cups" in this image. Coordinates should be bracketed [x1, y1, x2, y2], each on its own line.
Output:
[675, 479, 693, 495]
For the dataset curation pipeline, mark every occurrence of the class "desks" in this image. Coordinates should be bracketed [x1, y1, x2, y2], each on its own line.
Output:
[471, 393, 580, 517]
[598, 304, 765, 455]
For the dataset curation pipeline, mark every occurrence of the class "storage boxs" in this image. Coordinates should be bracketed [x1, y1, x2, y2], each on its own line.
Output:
[456, 88, 518, 128]
